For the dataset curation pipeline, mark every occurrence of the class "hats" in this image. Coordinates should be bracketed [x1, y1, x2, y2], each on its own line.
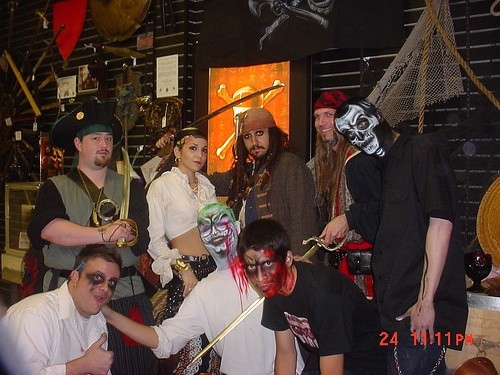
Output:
[314, 90, 348, 109]
[235, 108, 276, 136]
[48, 97, 126, 154]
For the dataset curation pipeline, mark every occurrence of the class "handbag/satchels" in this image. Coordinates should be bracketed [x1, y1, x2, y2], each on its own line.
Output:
[344, 250, 374, 275]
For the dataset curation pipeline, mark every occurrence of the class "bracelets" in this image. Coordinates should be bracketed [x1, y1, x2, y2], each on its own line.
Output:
[174, 258, 191, 271]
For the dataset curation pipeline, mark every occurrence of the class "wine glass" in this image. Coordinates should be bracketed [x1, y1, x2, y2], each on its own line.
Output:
[463, 251, 492, 294]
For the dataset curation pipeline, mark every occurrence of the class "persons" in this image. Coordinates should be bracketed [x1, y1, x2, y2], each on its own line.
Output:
[100, 203, 305, 375]
[28, 101, 155, 375]
[334, 90, 469, 375]
[237, 218, 378, 375]
[0, 244, 123, 375]
[156, 108, 322, 272]
[306, 89, 374, 300]
[146, 127, 226, 375]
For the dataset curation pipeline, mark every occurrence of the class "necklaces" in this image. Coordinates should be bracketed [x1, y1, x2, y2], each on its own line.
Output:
[76, 167, 103, 211]
